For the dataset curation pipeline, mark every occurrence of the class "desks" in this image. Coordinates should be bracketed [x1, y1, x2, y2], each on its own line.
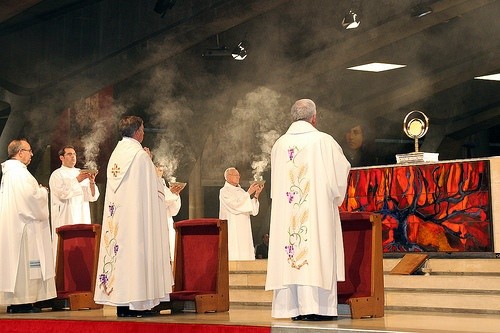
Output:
[338, 156, 499, 258]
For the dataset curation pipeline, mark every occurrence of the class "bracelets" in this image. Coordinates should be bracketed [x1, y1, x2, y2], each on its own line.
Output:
[254, 195, 257, 199]
[90, 181, 96, 184]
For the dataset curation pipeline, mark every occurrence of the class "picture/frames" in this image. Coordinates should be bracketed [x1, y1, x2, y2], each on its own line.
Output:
[339, 159, 495, 253]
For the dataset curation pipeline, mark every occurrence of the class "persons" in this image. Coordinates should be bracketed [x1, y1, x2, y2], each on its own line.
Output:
[93, 113, 174, 317]
[0, 140, 58, 313]
[256, 233, 269, 259]
[151, 157, 181, 270]
[218, 166, 264, 260]
[266, 97, 351, 320]
[334, 122, 373, 166]
[48, 145, 101, 275]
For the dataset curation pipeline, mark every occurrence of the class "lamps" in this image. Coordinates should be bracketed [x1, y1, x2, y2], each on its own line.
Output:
[340, 4, 363, 30]
[231, 38, 248, 60]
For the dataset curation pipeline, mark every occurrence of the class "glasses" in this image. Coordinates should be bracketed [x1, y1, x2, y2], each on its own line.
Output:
[63, 153, 76, 156]
[21, 149, 32, 153]
[228, 174, 241, 177]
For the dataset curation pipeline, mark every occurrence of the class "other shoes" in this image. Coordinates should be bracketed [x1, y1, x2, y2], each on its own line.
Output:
[7, 304, 40, 314]
[117, 306, 156, 318]
[292, 315, 333, 321]
